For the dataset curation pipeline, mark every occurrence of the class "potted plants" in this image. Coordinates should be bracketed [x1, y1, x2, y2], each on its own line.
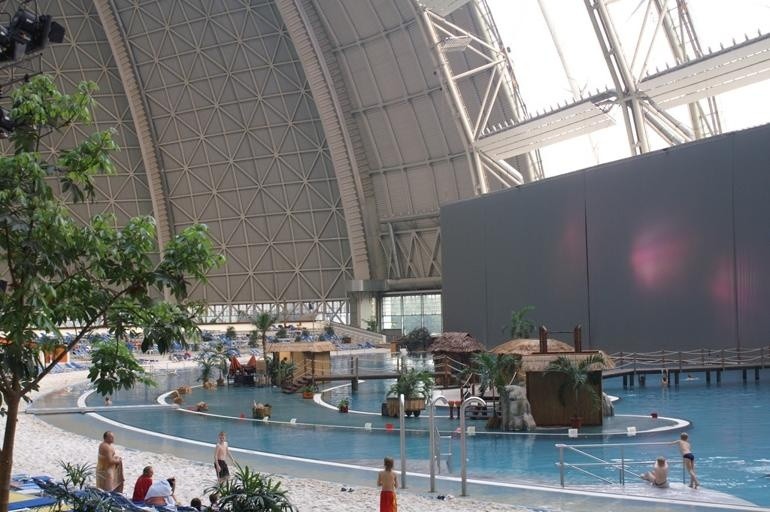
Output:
[543, 352, 614, 429]
[384, 364, 435, 412]
[337, 399, 349, 412]
[303, 386, 314, 399]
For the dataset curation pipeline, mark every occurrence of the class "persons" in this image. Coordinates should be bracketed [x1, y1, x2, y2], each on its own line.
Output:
[95, 431, 120, 494]
[640, 456, 670, 488]
[688, 374, 696, 381]
[253, 398, 272, 409]
[184, 345, 192, 360]
[639, 376, 644, 386]
[376, 455, 399, 512]
[661, 368, 669, 392]
[666, 432, 700, 489]
[213, 432, 234, 488]
[104, 396, 112, 406]
[651, 412, 659, 424]
[302, 383, 320, 393]
[132, 466, 224, 511]
